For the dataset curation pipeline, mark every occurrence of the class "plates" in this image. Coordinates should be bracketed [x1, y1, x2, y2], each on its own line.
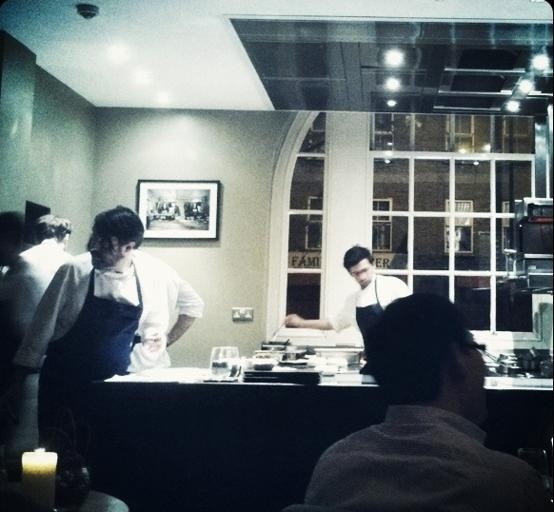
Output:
[252, 362, 274, 369]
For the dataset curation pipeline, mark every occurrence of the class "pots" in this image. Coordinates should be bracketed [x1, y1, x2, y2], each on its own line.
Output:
[481, 349, 521, 374]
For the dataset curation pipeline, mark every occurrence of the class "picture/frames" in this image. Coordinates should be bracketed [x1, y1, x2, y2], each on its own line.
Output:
[135, 176, 222, 244]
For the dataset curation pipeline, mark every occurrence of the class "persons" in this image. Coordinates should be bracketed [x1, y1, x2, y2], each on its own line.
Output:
[5, 205, 143, 452]
[0, 212, 77, 392]
[0, 210, 47, 455]
[0, 214, 76, 283]
[281, 244, 414, 353]
[86, 235, 201, 370]
[303, 293, 548, 511]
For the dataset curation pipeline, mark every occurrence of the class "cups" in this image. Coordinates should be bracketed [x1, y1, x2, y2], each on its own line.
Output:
[508, 367, 520, 377]
[209, 345, 240, 379]
[284, 352, 296, 361]
